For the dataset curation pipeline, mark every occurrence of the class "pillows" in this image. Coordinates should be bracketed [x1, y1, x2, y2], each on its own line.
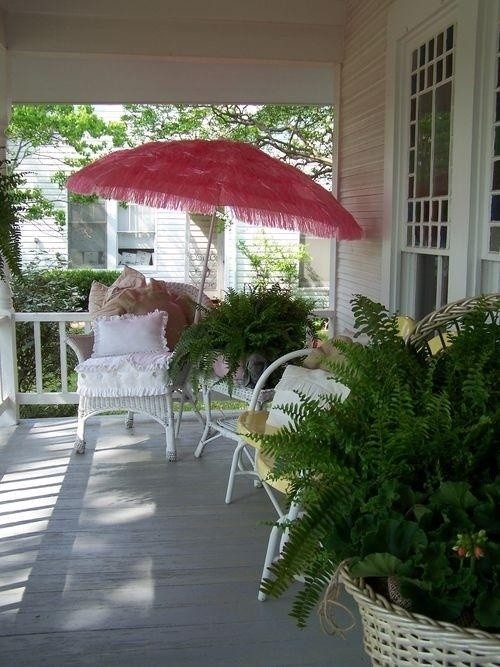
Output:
[88, 262, 196, 361]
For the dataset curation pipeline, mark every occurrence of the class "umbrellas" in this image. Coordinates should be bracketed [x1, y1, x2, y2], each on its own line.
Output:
[60, 140, 366, 438]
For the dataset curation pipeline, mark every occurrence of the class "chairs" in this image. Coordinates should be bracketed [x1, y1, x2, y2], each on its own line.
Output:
[63, 279, 216, 462]
[225, 313, 461, 603]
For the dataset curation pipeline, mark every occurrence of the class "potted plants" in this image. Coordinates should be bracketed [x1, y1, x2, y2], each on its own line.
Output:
[233, 288, 500, 667]
[159, 279, 330, 402]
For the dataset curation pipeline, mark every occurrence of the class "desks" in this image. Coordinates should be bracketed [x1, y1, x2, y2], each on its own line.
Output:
[195, 372, 276, 489]
